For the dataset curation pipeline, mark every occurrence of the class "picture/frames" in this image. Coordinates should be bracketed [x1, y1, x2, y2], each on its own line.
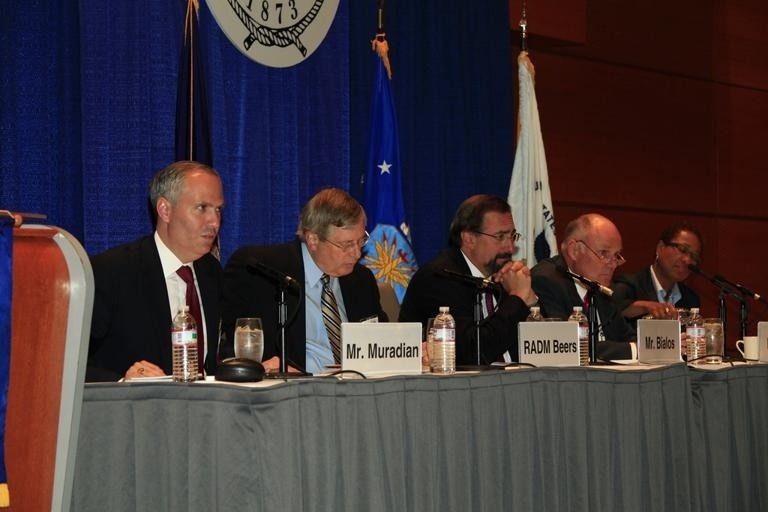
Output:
[76, 361, 768, 512]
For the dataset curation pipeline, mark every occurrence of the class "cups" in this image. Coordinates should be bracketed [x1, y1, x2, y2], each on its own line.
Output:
[477, 230, 520, 243]
[664, 240, 702, 264]
[312, 231, 370, 253]
[576, 239, 626, 266]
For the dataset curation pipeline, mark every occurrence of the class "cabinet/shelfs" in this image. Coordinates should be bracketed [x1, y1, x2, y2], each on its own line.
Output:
[568, 306, 590, 366]
[526, 306, 543, 320]
[169, 303, 198, 379]
[432, 306, 455, 374]
[642, 308, 697, 324]
[688, 307, 704, 368]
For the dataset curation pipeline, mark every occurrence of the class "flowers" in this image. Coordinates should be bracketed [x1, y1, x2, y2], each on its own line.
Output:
[235, 251, 299, 295]
[682, 260, 745, 307]
[554, 260, 616, 298]
[714, 269, 764, 306]
[427, 255, 497, 293]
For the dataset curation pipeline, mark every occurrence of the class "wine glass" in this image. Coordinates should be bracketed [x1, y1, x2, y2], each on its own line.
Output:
[139, 368, 146, 373]
[664, 307, 670, 313]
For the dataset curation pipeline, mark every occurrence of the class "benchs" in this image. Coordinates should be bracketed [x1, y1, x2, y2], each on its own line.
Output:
[506, 39, 559, 266]
[173, 0, 214, 160]
[358, 31, 420, 302]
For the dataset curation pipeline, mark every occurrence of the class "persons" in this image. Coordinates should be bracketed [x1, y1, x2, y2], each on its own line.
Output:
[529, 212, 705, 369]
[226, 185, 428, 373]
[87, 160, 299, 382]
[612, 217, 705, 318]
[400, 193, 547, 366]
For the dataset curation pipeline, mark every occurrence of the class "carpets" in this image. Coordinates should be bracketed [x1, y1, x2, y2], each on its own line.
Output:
[320, 274, 343, 363]
[585, 292, 591, 306]
[485, 288, 504, 361]
[176, 264, 206, 379]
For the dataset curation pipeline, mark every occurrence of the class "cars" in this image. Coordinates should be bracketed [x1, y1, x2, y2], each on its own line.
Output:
[233, 317, 265, 363]
[735, 336, 759, 363]
[702, 314, 725, 366]
